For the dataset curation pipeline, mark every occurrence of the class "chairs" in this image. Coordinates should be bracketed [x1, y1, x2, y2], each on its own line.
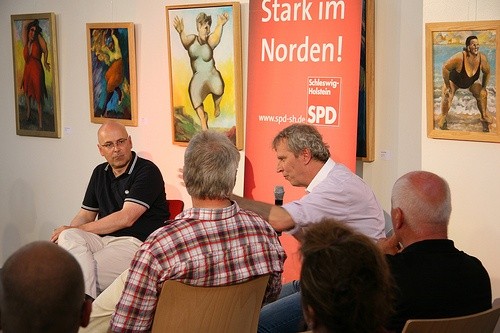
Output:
[151, 275, 271, 333]
[403, 298, 500, 333]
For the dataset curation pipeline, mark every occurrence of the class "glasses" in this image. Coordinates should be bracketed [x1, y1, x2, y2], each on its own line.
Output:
[101, 138, 129, 149]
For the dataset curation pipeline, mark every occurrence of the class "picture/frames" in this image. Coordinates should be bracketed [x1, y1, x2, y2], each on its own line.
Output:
[85, 22, 139, 128]
[165, 2, 244, 152]
[10, 12, 62, 139]
[357, 0, 376, 163]
[424, 20, 500, 144]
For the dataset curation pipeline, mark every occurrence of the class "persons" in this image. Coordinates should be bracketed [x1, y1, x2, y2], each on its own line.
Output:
[229, 124, 387, 333]
[50, 121, 168, 333]
[79, 131, 287, 333]
[376, 172, 492, 333]
[300, 218, 397, 333]
[0, 241, 92, 333]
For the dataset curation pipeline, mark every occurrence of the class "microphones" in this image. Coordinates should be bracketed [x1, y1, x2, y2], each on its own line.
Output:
[274, 186, 285, 236]
